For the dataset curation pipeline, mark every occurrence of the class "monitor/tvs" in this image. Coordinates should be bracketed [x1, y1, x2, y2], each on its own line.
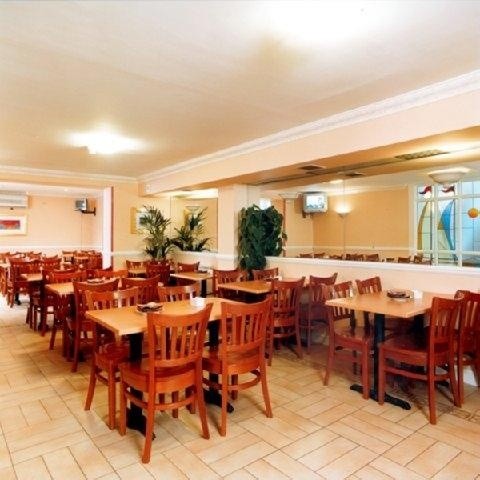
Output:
[73, 198, 89, 212]
[302, 191, 328, 213]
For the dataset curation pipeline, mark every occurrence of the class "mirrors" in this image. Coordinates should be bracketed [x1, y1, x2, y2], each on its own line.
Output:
[255, 159, 479, 269]
[170, 188, 218, 253]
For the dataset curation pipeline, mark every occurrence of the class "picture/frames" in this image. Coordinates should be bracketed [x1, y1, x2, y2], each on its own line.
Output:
[0, 213, 28, 236]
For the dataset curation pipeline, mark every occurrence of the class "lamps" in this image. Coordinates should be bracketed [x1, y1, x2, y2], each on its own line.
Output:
[428, 167, 470, 189]
[336, 179, 351, 218]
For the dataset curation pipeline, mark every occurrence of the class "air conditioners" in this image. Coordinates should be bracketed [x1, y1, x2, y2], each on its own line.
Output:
[0, 193, 28, 207]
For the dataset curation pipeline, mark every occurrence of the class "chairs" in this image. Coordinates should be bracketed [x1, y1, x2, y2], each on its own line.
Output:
[354, 276, 413, 383]
[320, 280, 394, 400]
[117, 302, 211, 463]
[296, 252, 433, 266]
[424, 288, 480, 404]
[188, 295, 273, 437]
[84, 287, 165, 431]
[157, 279, 233, 409]
[378, 296, 466, 425]
